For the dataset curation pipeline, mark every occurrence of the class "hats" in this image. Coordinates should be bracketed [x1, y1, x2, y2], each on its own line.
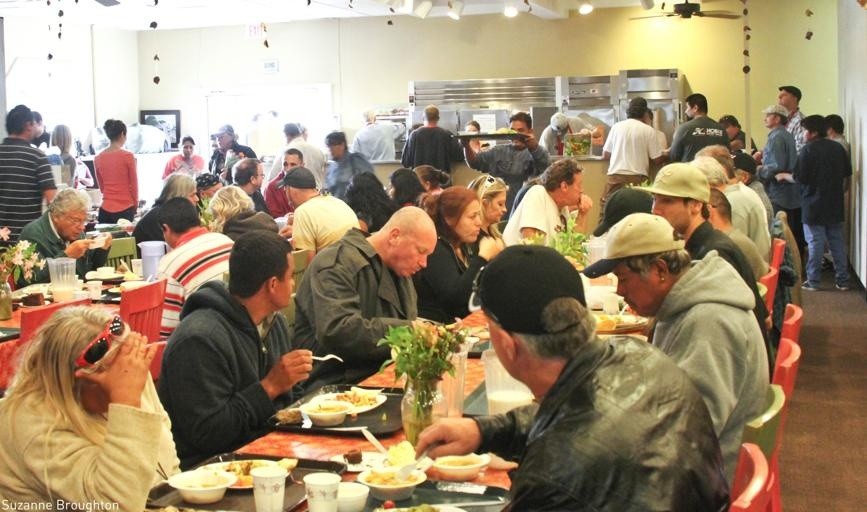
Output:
[582, 213, 686, 278]
[550, 113, 569, 136]
[778, 86, 801, 99]
[467, 244, 587, 335]
[729, 151, 757, 174]
[593, 186, 652, 237]
[762, 104, 789, 118]
[634, 162, 711, 204]
[275, 167, 316, 189]
[213, 124, 234, 136]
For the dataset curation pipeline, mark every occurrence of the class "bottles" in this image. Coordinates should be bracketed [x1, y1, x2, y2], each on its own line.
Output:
[589, 236, 610, 287]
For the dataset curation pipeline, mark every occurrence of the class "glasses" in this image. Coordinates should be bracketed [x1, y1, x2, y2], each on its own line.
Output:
[256, 173, 266, 178]
[480, 175, 496, 199]
[66, 216, 89, 226]
[75, 315, 125, 368]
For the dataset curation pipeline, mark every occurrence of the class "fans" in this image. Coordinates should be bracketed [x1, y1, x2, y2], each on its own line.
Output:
[626, 0, 741, 22]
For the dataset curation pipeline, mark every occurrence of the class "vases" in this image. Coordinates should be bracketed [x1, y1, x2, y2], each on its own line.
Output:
[401, 377, 449, 453]
[0, 272, 12, 318]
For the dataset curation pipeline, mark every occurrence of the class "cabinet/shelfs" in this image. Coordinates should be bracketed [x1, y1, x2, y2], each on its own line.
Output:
[368, 154, 612, 229]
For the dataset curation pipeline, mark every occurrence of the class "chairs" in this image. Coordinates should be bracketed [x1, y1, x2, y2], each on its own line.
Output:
[145, 342, 165, 382]
[281, 291, 298, 333]
[729, 236, 805, 512]
[288, 247, 317, 293]
[119, 279, 168, 341]
[20, 298, 93, 348]
[105, 237, 136, 271]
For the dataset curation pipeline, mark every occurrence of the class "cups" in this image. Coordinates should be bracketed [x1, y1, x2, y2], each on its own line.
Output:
[303, 472, 342, 512]
[46, 257, 77, 302]
[250, 468, 288, 512]
[131, 259, 143, 274]
[97, 266, 115, 278]
[88, 281, 103, 298]
[85, 232, 102, 250]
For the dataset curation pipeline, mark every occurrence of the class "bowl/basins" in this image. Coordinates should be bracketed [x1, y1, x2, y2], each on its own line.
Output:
[120, 281, 149, 292]
[357, 467, 427, 502]
[435, 454, 490, 481]
[298, 402, 352, 427]
[167, 470, 237, 504]
[337, 483, 370, 512]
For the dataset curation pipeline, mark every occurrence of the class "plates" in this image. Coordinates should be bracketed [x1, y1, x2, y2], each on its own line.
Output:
[310, 393, 388, 414]
[195, 460, 291, 490]
[332, 452, 384, 472]
[108, 288, 123, 294]
[85, 271, 124, 282]
[594, 315, 648, 328]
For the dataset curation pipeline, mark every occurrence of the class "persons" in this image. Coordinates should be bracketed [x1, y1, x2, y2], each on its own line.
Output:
[820, 114, 850, 273]
[756, 104, 809, 282]
[753, 86, 807, 164]
[775, 115, 852, 292]
[2, 93, 777, 512]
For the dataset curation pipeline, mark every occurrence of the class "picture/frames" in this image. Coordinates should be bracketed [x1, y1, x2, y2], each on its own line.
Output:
[140, 109, 181, 148]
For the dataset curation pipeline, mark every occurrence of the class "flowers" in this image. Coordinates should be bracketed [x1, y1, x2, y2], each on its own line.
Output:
[519, 210, 590, 269]
[0, 226, 47, 281]
[377, 319, 469, 396]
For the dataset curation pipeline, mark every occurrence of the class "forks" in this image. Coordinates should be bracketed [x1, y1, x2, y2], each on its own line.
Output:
[311, 354, 344, 364]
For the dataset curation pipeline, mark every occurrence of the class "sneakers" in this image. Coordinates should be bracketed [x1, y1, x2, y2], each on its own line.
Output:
[799, 280, 817, 292]
[836, 283, 850, 292]
